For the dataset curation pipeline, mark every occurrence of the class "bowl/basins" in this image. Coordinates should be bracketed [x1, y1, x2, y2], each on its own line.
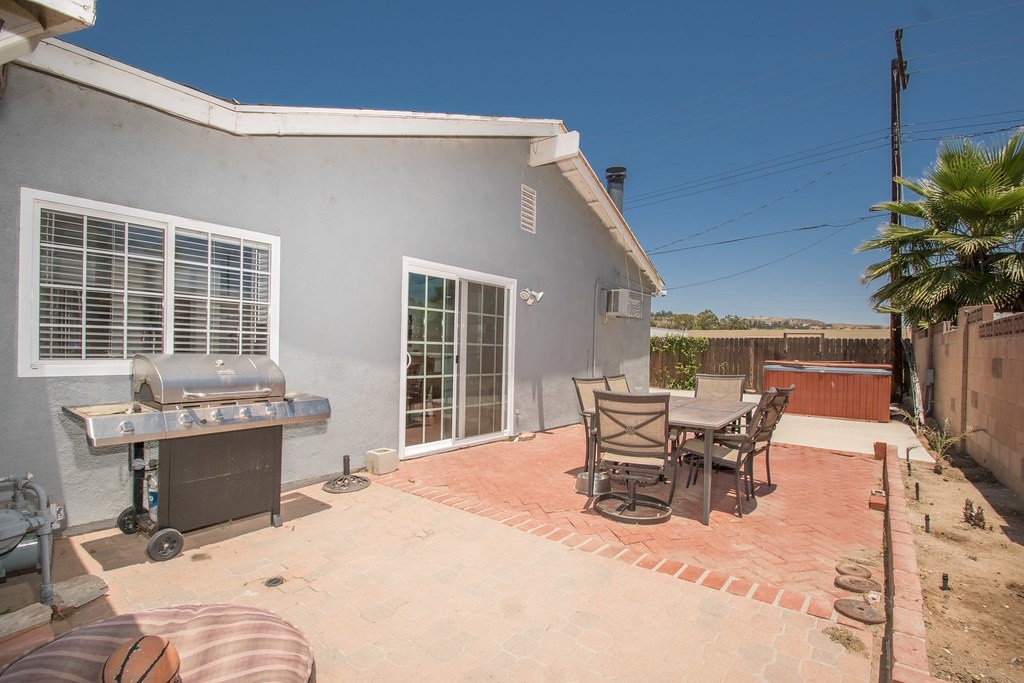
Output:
[573, 472, 614, 497]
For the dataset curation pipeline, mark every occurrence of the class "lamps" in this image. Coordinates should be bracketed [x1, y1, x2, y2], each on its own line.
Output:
[520, 287, 544, 305]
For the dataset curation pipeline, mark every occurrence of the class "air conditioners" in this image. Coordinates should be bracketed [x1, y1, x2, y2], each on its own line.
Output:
[604, 289, 642, 319]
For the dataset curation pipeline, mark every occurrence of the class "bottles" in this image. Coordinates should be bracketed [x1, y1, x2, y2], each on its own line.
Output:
[147, 471, 158, 523]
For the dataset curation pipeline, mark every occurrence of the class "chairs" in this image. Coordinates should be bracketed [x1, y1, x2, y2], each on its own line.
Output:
[668, 387, 778, 519]
[572, 377, 609, 472]
[694, 373, 746, 434]
[692, 384, 796, 499]
[602, 374, 630, 393]
[592, 388, 673, 525]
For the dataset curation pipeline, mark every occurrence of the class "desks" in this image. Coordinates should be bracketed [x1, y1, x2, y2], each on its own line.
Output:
[582, 395, 757, 526]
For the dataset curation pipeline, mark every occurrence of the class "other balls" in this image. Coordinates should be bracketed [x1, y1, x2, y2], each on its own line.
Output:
[99, 635, 182, 683]
